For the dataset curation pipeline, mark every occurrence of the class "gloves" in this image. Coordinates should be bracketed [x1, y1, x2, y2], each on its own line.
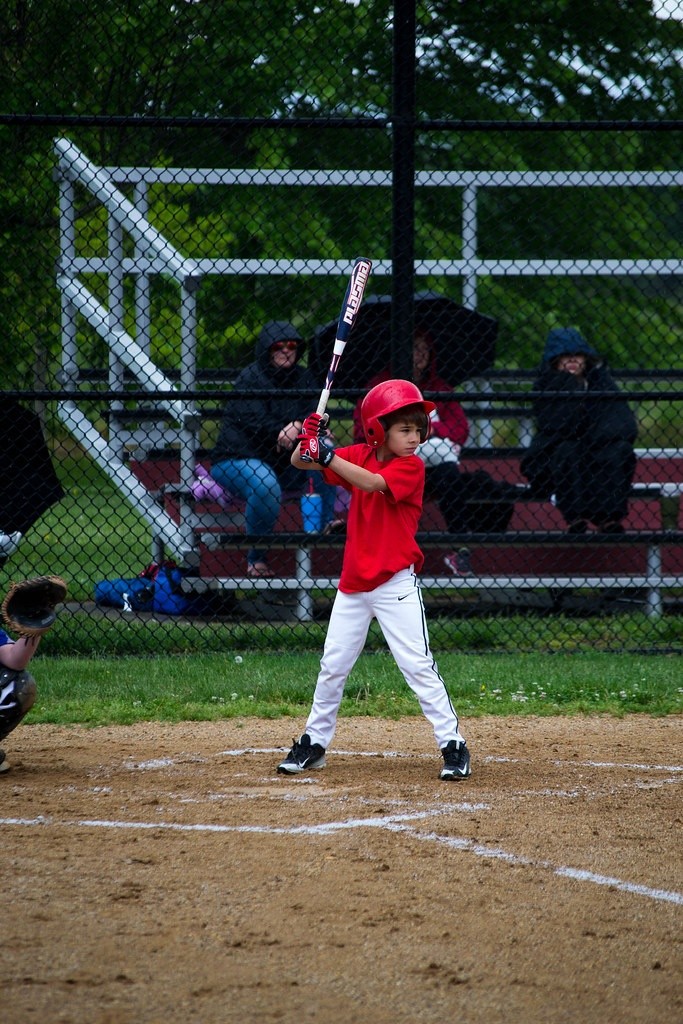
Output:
[301, 413, 330, 438]
[298, 432, 336, 467]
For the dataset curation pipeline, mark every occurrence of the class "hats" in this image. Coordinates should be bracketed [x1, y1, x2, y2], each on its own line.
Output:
[0, 532, 23, 557]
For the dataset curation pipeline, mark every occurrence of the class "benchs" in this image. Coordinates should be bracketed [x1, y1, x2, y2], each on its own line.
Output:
[79, 363, 682, 620]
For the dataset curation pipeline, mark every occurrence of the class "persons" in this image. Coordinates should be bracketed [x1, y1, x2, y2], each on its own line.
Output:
[277, 379, 472, 779]
[211, 322, 346, 578]
[520, 328, 637, 543]
[353, 334, 513, 575]
[0, 627, 41, 773]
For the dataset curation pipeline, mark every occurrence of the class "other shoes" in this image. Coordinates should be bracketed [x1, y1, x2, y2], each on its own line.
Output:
[444, 552, 475, 578]
[567, 520, 589, 533]
[599, 522, 624, 533]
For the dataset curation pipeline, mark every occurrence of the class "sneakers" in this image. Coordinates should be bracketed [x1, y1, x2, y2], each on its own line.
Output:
[439, 739, 472, 781]
[276, 734, 326, 775]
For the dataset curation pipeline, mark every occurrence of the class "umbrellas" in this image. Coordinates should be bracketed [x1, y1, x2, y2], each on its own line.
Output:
[0, 389, 66, 543]
[307, 289, 499, 406]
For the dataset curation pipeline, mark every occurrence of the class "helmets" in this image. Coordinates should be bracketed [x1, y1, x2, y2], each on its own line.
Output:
[360, 383, 437, 450]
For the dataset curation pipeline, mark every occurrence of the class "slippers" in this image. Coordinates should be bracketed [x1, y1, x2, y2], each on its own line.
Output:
[247, 563, 276, 579]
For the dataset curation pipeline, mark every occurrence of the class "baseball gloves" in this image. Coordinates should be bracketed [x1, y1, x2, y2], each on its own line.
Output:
[1, 574, 70, 649]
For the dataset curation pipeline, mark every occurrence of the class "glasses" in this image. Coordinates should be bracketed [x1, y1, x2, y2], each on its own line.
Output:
[273, 341, 298, 350]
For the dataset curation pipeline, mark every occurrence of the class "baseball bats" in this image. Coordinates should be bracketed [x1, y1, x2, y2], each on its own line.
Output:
[300, 255, 374, 463]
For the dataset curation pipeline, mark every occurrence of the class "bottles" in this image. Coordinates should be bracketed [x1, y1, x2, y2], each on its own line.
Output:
[300, 493, 322, 534]
